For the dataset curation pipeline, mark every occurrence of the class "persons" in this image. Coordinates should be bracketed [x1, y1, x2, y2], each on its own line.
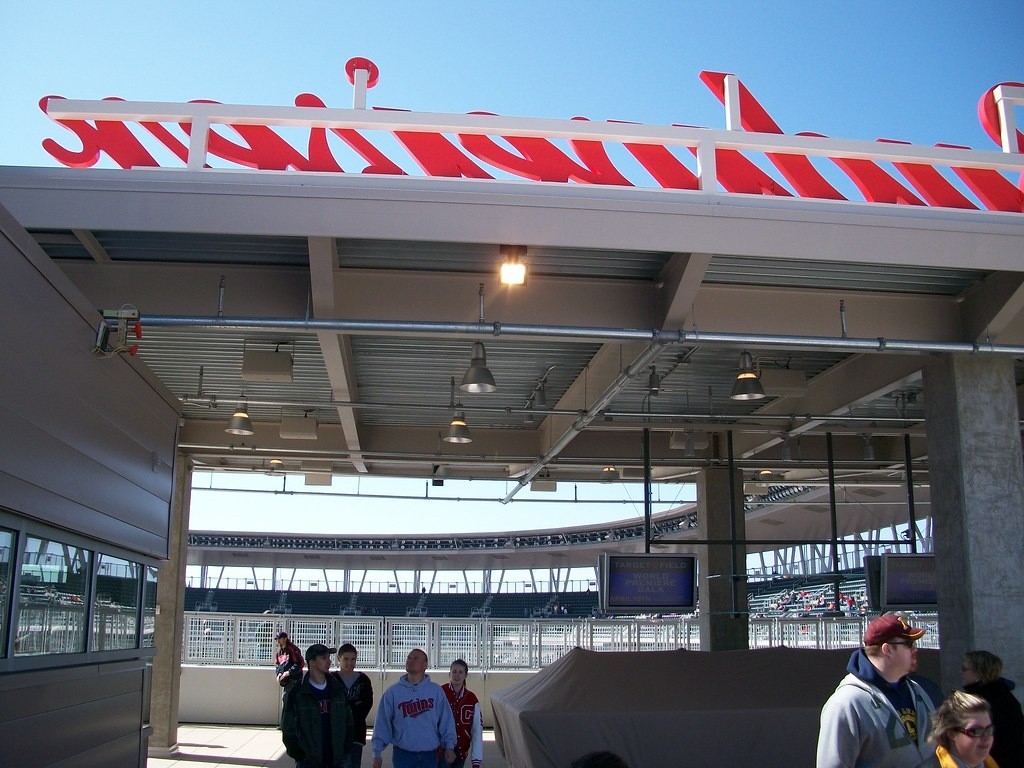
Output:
[548, 602, 568, 615]
[815, 615, 943, 768]
[912, 688, 998, 768]
[282, 644, 356, 768]
[750, 589, 870, 633]
[960, 651, 1024, 768]
[329, 643, 373, 768]
[370, 648, 457, 768]
[274, 632, 305, 730]
[439, 659, 483, 768]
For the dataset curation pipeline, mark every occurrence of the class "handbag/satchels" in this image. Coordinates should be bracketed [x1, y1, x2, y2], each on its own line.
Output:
[279, 674, 289, 686]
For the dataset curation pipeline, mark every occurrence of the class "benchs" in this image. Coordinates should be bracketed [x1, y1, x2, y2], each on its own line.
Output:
[748, 578, 868, 618]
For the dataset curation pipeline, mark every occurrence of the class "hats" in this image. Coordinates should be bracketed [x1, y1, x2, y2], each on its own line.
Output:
[274, 632, 288, 640]
[864, 615, 926, 646]
[305, 644, 337, 661]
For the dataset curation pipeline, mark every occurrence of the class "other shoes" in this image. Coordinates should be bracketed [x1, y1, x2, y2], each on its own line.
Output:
[277, 726, 281, 730]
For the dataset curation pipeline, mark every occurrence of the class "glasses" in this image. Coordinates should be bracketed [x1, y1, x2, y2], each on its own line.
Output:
[961, 664, 974, 672]
[953, 723, 994, 737]
[880, 638, 917, 649]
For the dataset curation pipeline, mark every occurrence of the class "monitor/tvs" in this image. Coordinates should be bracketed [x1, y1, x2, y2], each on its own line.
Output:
[597, 553, 697, 613]
[863, 553, 939, 610]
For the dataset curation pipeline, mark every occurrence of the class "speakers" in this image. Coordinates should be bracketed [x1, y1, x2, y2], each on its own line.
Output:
[279, 416, 317, 439]
[300, 460, 332, 471]
[241, 349, 293, 382]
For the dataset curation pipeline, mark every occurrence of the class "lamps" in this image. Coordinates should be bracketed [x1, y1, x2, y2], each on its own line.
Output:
[530, 480, 557, 492]
[649, 367, 660, 392]
[861, 436, 874, 460]
[459, 341, 496, 392]
[525, 402, 533, 423]
[668, 431, 710, 450]
[498, 245, 529, 287]
[682, 428, 697, 458]
[300, 459, 334, 471]
[224, 402, 254, 435]
[781, 441, 792, 461]
[537, 381, 546, 406]
[278, 406, 319, 440]
[243, 336, 294, 383]
[729, 352, 766, 400]
[304, 474, 333, 486]
[742, 482, 769, 496]
[618, 467, 655, 479]
[440, 404, 473, 444]
